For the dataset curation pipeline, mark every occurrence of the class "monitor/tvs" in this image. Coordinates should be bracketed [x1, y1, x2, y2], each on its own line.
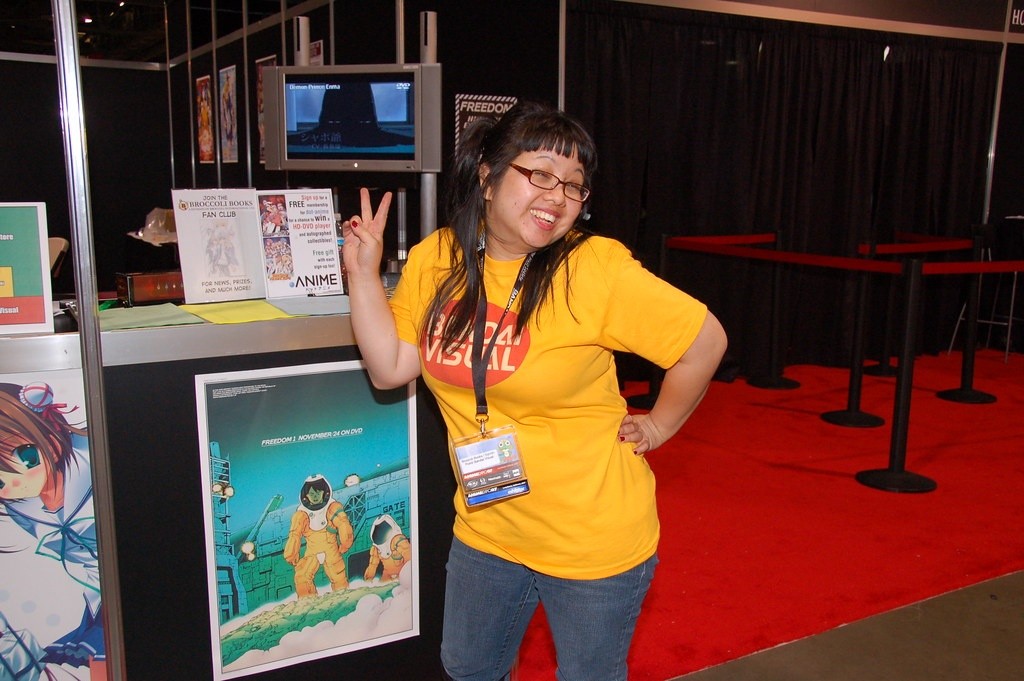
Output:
[262, 63, 441, 173]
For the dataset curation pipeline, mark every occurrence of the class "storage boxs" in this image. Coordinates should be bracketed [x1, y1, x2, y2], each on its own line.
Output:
[117, 271, 184, 309]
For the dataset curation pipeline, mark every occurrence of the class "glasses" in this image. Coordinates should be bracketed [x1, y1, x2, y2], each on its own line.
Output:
[510, 162, 590, 201]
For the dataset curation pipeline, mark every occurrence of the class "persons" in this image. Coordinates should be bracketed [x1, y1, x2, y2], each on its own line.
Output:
[341, 101, 730, 681]
